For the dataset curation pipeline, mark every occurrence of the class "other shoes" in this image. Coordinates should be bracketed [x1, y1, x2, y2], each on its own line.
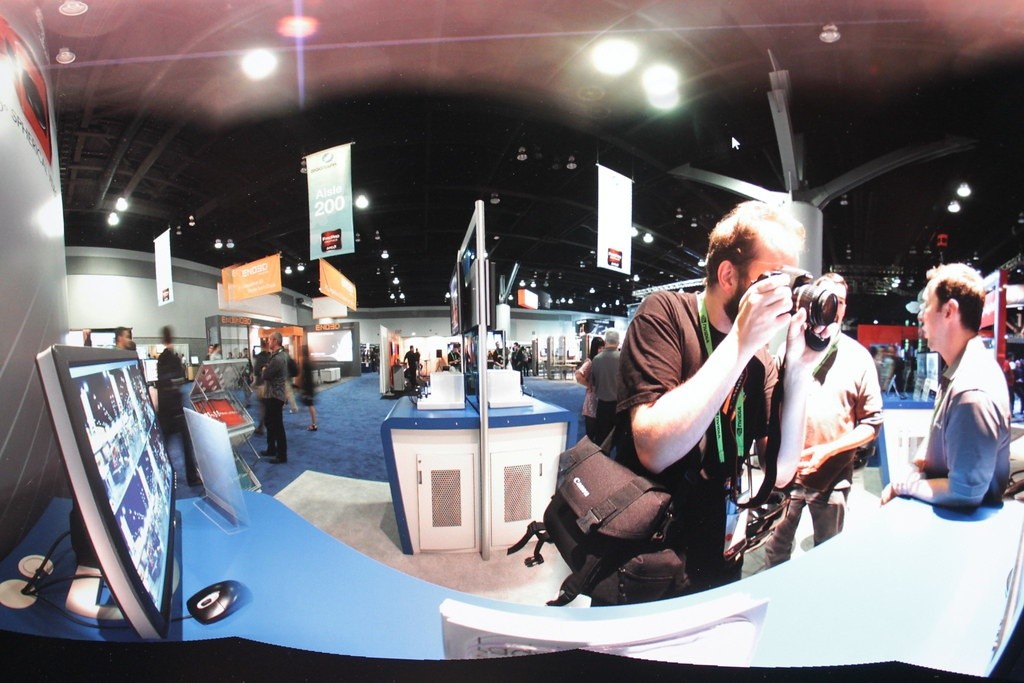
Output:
[289, 408, 298, 414]
[260, 448, 277, 457]
[188, 476, 203, 487]
[306, 424, 318, 431]
[254, 426, 265, 435]
[269, 456, 288, 464]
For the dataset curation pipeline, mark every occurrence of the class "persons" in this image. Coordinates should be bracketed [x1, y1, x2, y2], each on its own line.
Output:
[225, 348, 253, 388]
[155, 327, 202, 487]
[370, 347, 378, 372]
[575, 330, 621, 457]
[512, 342, 529, 385]
[404, 345, 417, 384]
[448, 344, 461, 367]
[299, 345, 318, 431]
[869, 345, 904, 392]
[763, 273, 884, 569]
[255, 332, 298, 464]
[1004, 352, 1024, 414]
[880, 263, 1012, 508]
[492, 341, 503, 369]
[205, 344, 222, 360]
[113, 327, 130, 349]
[615, 200, 840, 594]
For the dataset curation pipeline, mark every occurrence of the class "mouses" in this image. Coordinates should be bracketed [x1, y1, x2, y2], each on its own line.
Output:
[186, 579, 240, 625]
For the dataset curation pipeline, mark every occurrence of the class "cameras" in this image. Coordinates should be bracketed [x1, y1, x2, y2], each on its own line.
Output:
[753, 264, 838, 327]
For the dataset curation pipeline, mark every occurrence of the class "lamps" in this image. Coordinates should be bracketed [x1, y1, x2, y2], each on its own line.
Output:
[516, 146, 527, 161]
[355, 193, 406, 301]
[490, 192, 500, 204]
[819, 23, 841, 43]
[957, 181, 972, 197]
[567, 154, 577, 169]
[175, 214, 305, 274]
[947, 200, 961, 213]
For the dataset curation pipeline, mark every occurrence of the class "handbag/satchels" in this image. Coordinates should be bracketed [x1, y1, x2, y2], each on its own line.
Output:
[506, 432, 692, 607]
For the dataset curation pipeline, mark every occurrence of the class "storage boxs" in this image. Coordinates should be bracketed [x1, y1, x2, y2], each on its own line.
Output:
[430, 371, 463, 403]
[486, 369, 521, 403]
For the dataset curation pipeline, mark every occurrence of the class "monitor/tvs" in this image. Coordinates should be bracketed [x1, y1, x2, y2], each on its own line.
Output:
[448, 225, 496, 337]
[32, 343, 178, 640]
